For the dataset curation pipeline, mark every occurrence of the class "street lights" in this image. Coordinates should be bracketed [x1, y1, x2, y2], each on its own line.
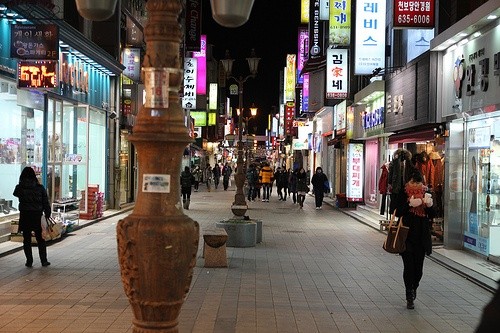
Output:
[216, 47, 263, 248]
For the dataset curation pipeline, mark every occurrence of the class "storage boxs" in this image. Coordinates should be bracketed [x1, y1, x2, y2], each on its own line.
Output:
[80, 184, 104, 219]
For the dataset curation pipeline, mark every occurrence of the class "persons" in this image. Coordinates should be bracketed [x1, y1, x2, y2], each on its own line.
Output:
[246, 164, 261, 202]
[12, 167, 52, 267]
[180, 166, 196, 210]
[270, 167, 299, 204]
[296, 167, 307, 208]
[311, 167, 328, 210]
[390, 171, 437, 310]
[213, 163, 222, 190]
[258, 163, 273, 202]
[192, 166, 202, 192]
[190, 160, 195, 170]
[205, 163, 213, 192]
[39, 64, 54, 84]
[469, 157, 478, 214]
[222, 162, 233, 191]
[473, 280, 500, 333]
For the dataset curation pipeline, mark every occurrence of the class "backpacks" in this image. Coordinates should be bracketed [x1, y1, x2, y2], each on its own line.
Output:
[181, 175, 193, 187]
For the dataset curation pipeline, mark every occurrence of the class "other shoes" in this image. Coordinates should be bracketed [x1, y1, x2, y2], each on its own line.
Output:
[316, 206, 322, 210]
[25, 263, 32, 267]
[42, 262, 50, 266]
[261, 199, 269, 202]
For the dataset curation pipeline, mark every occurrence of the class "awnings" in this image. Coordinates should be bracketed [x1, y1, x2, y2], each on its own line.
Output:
[327, 134, 345, 146]
[388, 128, 443, 145]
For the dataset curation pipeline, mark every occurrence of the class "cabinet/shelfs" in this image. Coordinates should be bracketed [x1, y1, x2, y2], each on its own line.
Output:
[50, 202, 78, 226]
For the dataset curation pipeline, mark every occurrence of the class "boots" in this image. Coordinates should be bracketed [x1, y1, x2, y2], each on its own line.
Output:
[406, 289, 417, 308]
[183, 198, 190, 210]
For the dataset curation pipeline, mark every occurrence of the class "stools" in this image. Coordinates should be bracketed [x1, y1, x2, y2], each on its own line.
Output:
[378, 219, 397, 233]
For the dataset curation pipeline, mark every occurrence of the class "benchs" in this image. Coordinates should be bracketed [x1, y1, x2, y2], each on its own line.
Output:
[202, 227, 228, 267]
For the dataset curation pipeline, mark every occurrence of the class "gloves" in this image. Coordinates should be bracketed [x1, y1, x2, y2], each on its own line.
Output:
[423, 198, 433, 208]
[410, 196, 422, 207]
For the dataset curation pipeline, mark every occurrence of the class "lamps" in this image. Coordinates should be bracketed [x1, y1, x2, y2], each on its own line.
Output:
[101, 102, 117, 119]
[372, 66, 405, 76]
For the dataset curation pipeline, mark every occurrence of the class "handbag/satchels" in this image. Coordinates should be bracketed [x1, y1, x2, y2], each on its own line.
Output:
[46, 217, 66, 240]
[382, 210, 409, 253]
[304, 186, 310, 193]
[41, 215, 51, 241]
